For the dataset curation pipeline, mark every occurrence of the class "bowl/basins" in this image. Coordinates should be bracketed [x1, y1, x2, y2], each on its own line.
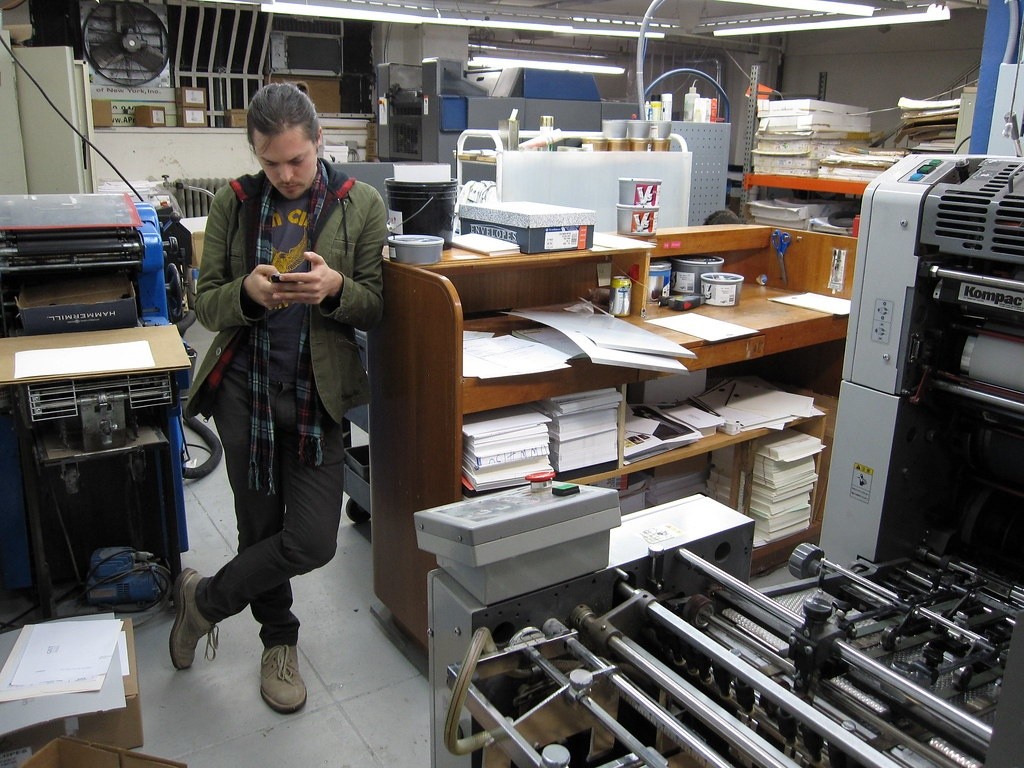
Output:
[701, 273, 744, 306]
[618, 179, 662, 207]
[648, 263, 671, 303]
[670, 256, 724, 295]
[616, 203, 659, 236]
[388, 234, 444, 263]
[583, 119, 672, 151]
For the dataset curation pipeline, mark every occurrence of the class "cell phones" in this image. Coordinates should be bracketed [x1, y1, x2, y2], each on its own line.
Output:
[275, 261, 312, 284]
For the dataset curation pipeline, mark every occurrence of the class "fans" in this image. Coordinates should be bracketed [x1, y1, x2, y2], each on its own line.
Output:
[82, 0, 171, 87]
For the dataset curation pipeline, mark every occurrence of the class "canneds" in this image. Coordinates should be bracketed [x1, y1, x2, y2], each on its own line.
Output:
[608, 276, 632, 316]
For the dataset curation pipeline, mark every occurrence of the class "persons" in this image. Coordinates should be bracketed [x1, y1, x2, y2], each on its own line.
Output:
[168, 81, 387, 715]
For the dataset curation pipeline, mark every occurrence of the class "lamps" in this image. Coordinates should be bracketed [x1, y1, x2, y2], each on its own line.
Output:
[468, 40, 627, 74]
[695, 0, 951, 37]
[261, 0, 686, 40]
[715, 0, 909, 17]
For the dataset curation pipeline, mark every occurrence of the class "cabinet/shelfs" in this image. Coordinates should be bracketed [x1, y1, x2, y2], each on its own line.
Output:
[741, 64, 977, 238]
[370, 223, 859, 654]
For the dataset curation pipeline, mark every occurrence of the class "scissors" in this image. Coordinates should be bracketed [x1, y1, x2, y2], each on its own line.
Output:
[772, 229, 792, 286]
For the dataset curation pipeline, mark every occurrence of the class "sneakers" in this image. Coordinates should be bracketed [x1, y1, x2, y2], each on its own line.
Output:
[169, 567, 219, 670]
[260, 642, 307, 715]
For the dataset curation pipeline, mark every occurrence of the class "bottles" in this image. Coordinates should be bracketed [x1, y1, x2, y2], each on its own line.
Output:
[650, 102, 661, 121]
[661, 94, 673, 120]
[540, 116, 554, 135]
[684, 87, 700, 121]
[644, 101, 651, 121]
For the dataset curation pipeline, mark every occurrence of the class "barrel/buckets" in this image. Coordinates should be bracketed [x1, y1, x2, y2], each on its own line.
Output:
[385, 177, 458, 250]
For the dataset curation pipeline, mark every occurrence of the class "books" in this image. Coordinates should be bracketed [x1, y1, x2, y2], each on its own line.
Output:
[818, 148, 906, 182]
[528, 387, 623, 472]
[624, 405, 726, 466]
[587, 453, 708, 515]
[746, 198, 860, 234]
[898, 96, 962, 153]
[462, 405, 554, 491]
[644, 368, 706, 404]
[451, 233, 520, 257]
[706, 428, 826, 542]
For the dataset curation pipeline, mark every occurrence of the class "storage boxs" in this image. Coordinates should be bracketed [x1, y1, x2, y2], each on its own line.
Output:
[0, 617, 144, 768]
[366, 139, 378, 156]
[22, 735, 188, 768]
[135, 105, 165, 127]
[92, 99, 113, 127]
[367, 122, 379, 140]
[224, 109, 248, 128]
[176, 108, 208, 128]
[176, 87, 207, 108]
[458, 200, 595, 254]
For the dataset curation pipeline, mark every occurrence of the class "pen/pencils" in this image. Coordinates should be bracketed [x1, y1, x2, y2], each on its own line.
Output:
[578, 296, 614, 318]
